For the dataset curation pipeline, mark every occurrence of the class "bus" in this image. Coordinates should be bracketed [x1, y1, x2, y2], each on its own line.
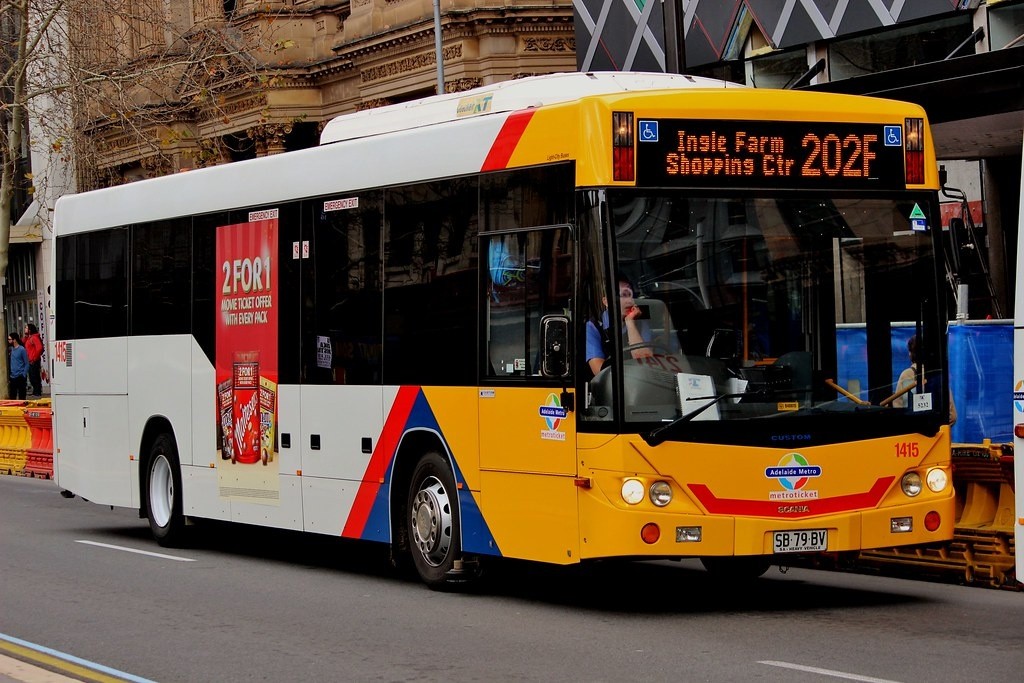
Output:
[49, 70, 971, 588]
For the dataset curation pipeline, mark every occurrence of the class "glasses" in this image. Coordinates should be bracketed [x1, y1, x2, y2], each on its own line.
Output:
[8, 338, 12, 340]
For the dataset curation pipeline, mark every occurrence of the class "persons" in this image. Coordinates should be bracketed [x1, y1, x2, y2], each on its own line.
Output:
[893, 336, 957, 426]
[587, 280, 652, 375]
[8, 323, 43, 399]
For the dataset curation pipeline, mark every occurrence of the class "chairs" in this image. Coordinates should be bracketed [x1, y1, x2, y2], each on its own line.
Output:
[635, 299, 681, 356]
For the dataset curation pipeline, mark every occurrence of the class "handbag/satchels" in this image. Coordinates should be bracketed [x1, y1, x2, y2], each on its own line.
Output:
[902, 367, 917, 405]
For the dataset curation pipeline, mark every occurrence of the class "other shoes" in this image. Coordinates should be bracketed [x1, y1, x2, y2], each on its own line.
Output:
[29, 392, 41, 396]
